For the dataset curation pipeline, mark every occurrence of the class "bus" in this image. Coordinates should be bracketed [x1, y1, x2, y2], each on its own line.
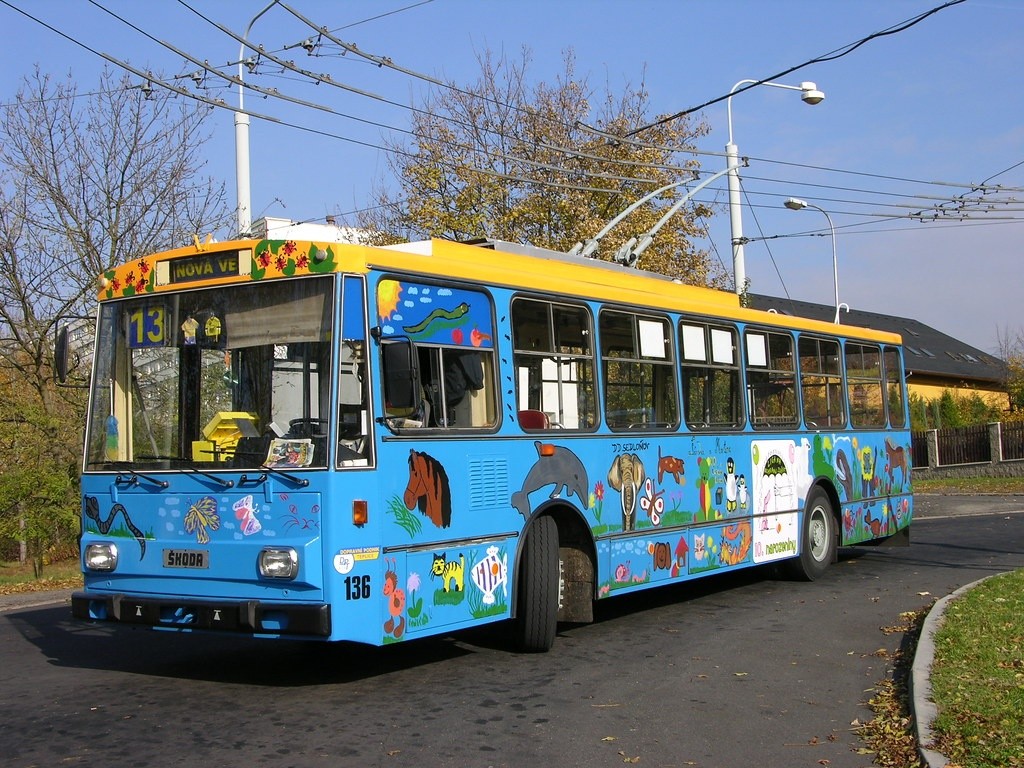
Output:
[55, 158, 915, 652]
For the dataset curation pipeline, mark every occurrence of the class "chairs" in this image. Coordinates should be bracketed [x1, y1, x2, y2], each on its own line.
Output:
[519, 408, 553, 431]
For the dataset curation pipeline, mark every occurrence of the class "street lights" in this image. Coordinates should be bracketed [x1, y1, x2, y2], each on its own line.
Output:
[783, 197, 840, 327]
[726, 79, 825, 296]
